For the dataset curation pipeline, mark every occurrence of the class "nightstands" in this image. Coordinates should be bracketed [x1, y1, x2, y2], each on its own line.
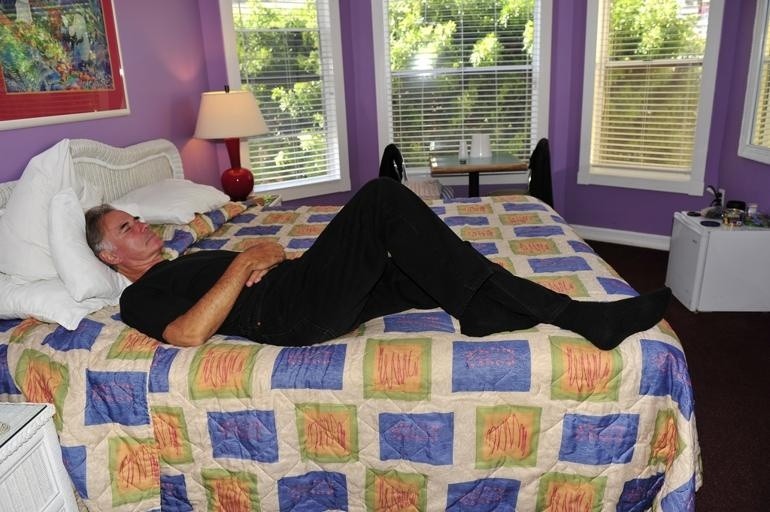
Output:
[1, 400, 81, 511]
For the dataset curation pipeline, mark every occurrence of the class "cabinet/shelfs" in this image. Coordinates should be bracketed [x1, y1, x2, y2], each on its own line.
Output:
[668, 211, 770, 314]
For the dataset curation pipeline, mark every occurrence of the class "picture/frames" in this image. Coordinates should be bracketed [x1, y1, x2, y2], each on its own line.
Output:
[0, 1, 131, 132]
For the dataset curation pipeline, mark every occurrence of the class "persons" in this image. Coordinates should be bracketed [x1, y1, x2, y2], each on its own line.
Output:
[85, 177, 671, 350]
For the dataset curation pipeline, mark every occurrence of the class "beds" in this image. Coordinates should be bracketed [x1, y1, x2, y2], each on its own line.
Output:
[0, 136, 705, 511]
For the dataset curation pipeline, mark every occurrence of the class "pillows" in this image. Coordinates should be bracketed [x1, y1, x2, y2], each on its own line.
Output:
[108, 176, 231, 225]
[1, 137, 134, 331]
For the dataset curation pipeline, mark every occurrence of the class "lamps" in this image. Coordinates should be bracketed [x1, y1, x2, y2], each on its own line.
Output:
[193, 82, 272, 202]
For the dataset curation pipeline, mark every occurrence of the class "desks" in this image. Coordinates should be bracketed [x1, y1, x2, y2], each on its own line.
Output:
[428, 150, 528, 197]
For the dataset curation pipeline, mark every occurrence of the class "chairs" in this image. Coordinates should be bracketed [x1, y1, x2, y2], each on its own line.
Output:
[486, 135, 554, 208]
[378, 143, 443, 199]
